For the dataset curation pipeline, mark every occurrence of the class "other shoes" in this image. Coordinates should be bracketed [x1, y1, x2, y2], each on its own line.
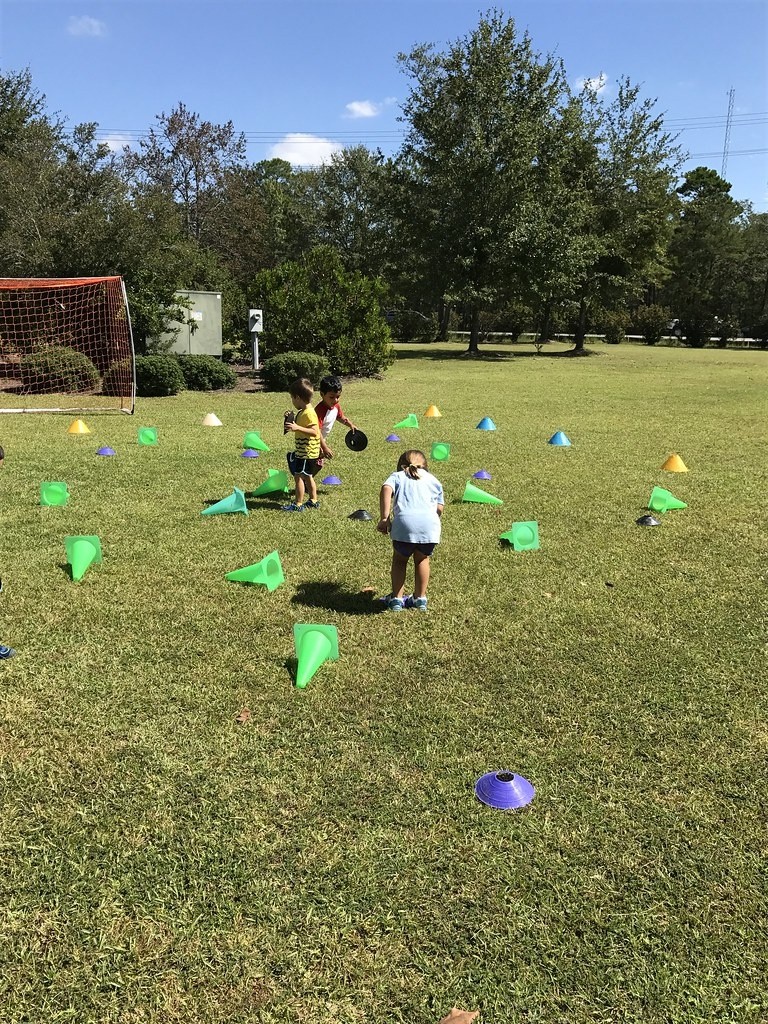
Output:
[0, 645, 15, 659]
[287, 452, 295, 476]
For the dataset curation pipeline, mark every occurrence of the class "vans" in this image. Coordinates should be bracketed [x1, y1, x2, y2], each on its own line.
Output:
[387, 311, 430, 323]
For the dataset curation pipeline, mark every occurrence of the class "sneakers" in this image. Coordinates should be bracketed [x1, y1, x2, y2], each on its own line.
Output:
[303, 498, 321, 509]
[384, 592, 403, 612]
[405, 593, 427, 611]
[282, 502, 305, 512]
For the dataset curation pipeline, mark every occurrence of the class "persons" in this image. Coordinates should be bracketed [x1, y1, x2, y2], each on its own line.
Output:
[376, 449, 445, 611]
[279, 377, 322, 511]
[287, 376, 358, 477]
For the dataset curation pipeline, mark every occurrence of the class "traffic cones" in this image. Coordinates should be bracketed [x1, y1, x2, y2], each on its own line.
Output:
[64, 535, 102, 582]
[67, 419, 89, 434]
[294, 624, 339, 688]
[422, 405, 443, 417]
[243, 430, 270, 451]
[41, 483, 70, 506]
[475, 417, 497, 431]
[226, 549, 285, 592]
[661, 454, 690, 472]
[392, 414, 418, 428]
[461, 480, 504, 505]
[430, 441, 450, 461]
[499, 521, 539, 552]
[137, 428, 158, 445]
[547, 431, 573, 445]
[647, 486, 687, 513]
[203, 412, 221, 426]
[251, 469, 289, 497]
[200, 487, 249, 517]
